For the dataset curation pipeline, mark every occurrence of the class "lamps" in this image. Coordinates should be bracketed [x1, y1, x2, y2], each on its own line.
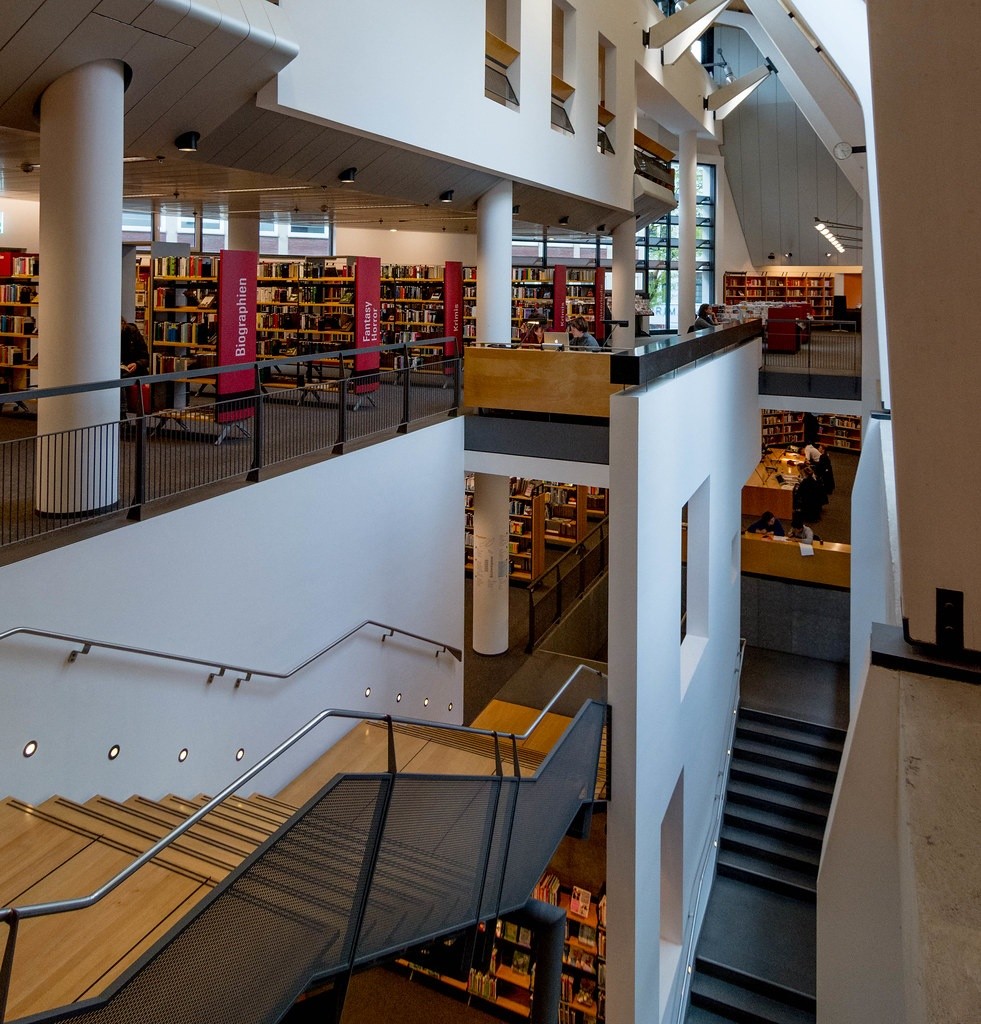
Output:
[440, 192, 454, 204]
[512, 205, 520, 216]
[768, 252, 776, 260]
[597, 224, 606, 232]
[658, 0, 691, 13]
[559, 217, 569, 225]
[814, 217, 863, 258]
[175, 131, 202, 152]
[338, 168, 357, 183]
[784, 252, 792, 257]
[705, 47, 737, 87]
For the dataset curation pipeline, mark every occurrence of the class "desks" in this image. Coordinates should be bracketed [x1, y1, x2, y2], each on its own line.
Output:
[742, 447, 807, 520]
[741, 531, 851, 590]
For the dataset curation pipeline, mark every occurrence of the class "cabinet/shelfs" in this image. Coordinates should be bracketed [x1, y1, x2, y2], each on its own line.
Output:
[566, 268, 606, 339]
[544, 480, 588, 546]
[723, 274, 835, 322]
[257, 254, 382, 411]
[588, 487, 608, 518]
[762, 408, 862, 452]
[396, 890, 606, 1024]
[150, 251, 258, 446]
[1, 276, 40, 369]
[466, 266, 566, 338]
[465, 490, 544, 583]
[381, 262, 463, 389]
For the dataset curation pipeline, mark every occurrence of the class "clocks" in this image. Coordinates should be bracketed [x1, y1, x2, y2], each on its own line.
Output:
[834, 143, 851, 159]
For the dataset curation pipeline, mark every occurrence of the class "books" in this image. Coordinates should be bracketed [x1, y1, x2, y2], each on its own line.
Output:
[135, 256, 220, 379]
[465, 476, 605, 575]
[463, 266, 476, 357]
[761, 408, 860, 448]
[0, 251, 39, 392]
[380, 264, 444, 372]
[468, 870, 607, 1024]
[120, 365, 129, 372]
[511, 268, 555, 339]
[566, 268, 596, 334]
[725, 279, 831, 317]
[256, 260, 357, 360]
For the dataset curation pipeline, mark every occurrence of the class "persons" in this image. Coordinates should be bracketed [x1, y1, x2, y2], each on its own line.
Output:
[694, 304, 719, 332]
[569, 316, 601, 352]
[787, 519, 813, 544]
[521, 313, 547, 349]
[792, 412, 827, 525]
[120, 315, 150, 441]
[605, 298, 612, 347]
[747, 511, 786, 537]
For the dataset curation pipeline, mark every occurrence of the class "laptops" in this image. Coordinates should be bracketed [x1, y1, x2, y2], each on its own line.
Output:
[544, 332, 569, 350]
[775, 473, 788, 485]
